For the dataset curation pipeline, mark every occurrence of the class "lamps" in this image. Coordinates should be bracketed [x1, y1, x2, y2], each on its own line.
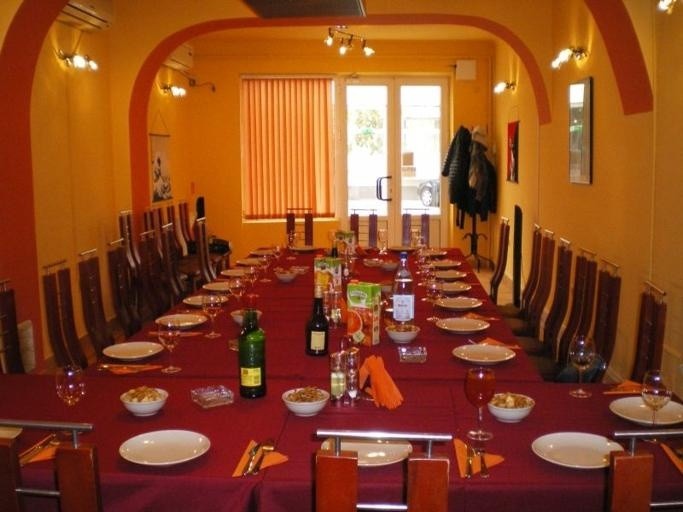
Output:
[325, 25, 375, 58]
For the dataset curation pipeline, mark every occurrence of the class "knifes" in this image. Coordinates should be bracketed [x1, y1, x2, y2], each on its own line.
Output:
[463, 442, 477, 480]
[243, 436, 260, 476]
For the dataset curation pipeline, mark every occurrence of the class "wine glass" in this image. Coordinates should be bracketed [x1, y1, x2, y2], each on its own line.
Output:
[156, 230, 297, 375]
[567, 335, 596, 402]
[377, 227, 444, 332]
[55, 368, 89, 435]
[642, 372, 671, 429]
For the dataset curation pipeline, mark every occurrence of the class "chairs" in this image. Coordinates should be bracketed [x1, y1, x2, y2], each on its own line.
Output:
[284, 206, 432, 249]
[0, 201, 231, 361]
[487, 216, 669, 383]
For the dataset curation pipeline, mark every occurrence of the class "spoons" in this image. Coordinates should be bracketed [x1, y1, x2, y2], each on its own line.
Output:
[256, 433, 278, 479]
[474, 440, 493, 485]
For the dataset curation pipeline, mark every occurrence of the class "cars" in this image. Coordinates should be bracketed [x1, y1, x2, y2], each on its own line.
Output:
[417, 180, 439, 208]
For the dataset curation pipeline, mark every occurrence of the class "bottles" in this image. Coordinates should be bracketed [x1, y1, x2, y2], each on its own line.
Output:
[304, 228, 414, 410]
[241, 296, 268, 397]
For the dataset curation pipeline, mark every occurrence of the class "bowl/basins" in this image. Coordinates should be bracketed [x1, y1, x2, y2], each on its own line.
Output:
[488, 385, 538, 424]
[229, 309, 262, 327]
[283, 383, 329, 416]
[385, 321, 422, 346]
[119, 384, 168, 418]
[273, 270, 296, 284]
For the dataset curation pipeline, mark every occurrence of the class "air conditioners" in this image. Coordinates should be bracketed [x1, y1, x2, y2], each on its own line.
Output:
[55, 0, 114, 35]
[162, 42, 194, 73]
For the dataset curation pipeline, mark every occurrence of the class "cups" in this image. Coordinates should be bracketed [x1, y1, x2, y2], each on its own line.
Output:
[460, 368, 496, 441]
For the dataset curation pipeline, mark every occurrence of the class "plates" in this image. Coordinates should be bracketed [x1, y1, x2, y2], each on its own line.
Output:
[319, 430, 414, 468]
[416, 236, 519, 369]
[614, 394, 683, 429]
[117, 428, 213, 468]
[528, 431, 628, 470]
[101, 223, 315, 360]
